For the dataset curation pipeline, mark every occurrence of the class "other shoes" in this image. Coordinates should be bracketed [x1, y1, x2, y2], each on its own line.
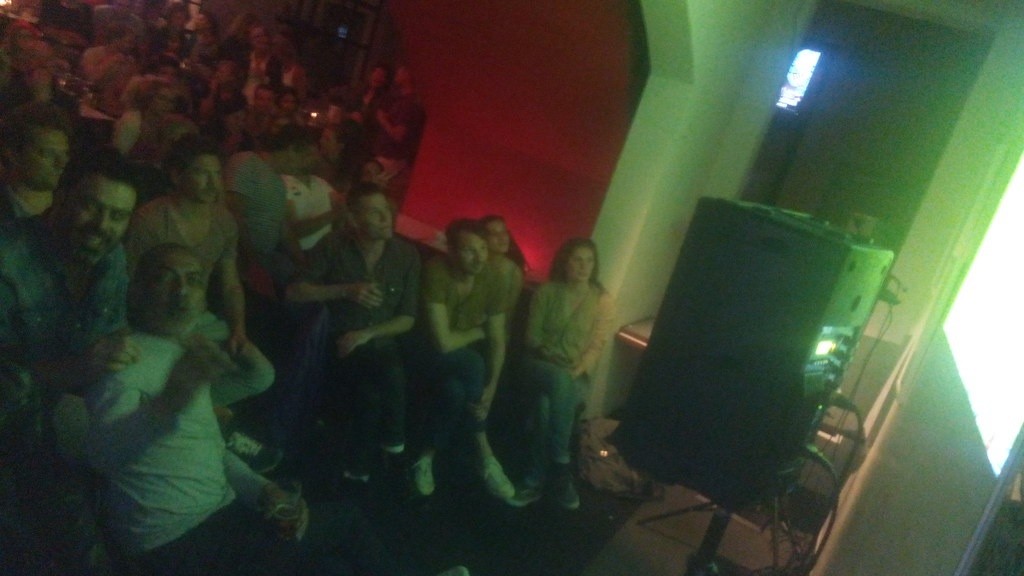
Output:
[379, 441, 405, 453]
[339, 445, 370, 482]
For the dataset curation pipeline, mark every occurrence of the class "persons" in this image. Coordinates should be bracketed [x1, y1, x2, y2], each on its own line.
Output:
[509, 239, 615, 509]
[84, 244, 308, 576]
[0, 0, 422, 576]
[414, 214, 525, 501]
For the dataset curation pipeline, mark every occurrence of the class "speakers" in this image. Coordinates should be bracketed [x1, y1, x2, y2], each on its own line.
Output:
[618, 196, 894, 533]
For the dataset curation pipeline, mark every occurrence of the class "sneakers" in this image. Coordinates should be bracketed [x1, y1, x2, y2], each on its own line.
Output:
[223, 428, 283, 474]
[551, 463, 580, 511]
[477, 454, 515, 499]
[412, 456, 435, 496]
[506, 477, 542, 507]
